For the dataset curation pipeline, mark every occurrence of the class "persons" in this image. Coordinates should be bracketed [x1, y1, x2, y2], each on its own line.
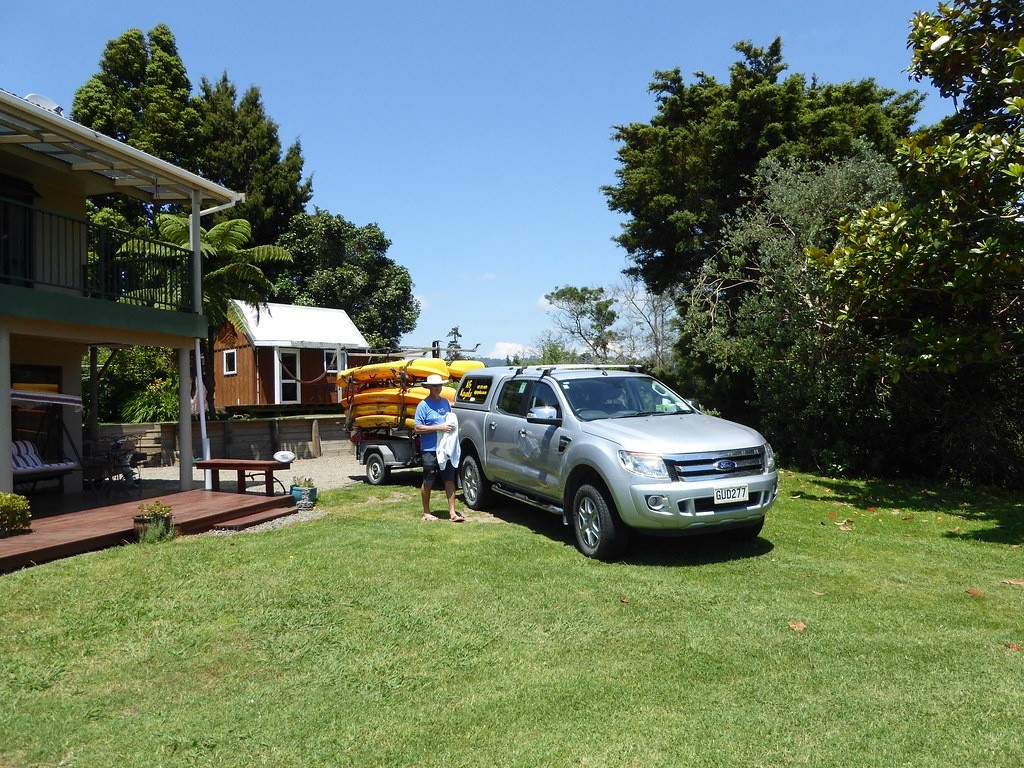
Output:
[413, 374, 466, 521]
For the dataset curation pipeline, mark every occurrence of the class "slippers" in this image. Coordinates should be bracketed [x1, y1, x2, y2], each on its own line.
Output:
[421, 514, 439, 521]
[448, 515, 466, 523]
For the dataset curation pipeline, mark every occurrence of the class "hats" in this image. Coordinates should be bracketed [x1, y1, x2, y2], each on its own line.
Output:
[422, 374, 450, 389]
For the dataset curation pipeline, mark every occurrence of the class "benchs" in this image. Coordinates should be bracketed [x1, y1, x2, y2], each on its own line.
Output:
[12, 440, 79, 500]
[195, 459, 290, 496]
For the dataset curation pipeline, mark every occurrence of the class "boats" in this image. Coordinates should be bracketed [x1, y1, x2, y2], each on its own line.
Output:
[336, 358, 485, 444]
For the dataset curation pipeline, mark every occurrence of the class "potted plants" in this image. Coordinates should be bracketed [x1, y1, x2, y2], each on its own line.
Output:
[290, 476, 317, 503]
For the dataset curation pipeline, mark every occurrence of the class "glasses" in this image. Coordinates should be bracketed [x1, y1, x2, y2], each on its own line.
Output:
[432, 385, 442, 387]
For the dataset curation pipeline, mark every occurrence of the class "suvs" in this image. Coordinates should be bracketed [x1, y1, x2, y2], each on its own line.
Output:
[452, 364, 777, 558]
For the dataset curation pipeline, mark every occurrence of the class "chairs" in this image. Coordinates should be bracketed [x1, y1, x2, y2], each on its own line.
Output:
[600, 383, 627, 413]
[92, 434, 144, 495]
